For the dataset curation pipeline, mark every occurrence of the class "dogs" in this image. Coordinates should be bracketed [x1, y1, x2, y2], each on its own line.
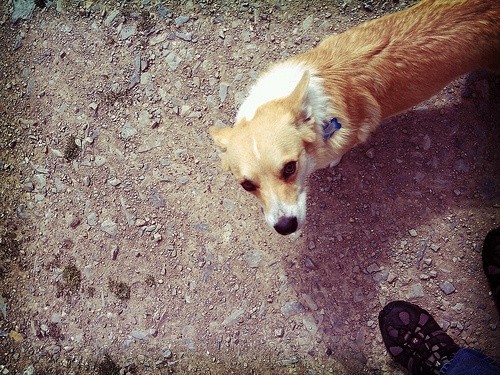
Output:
[209, 0, 500, 236]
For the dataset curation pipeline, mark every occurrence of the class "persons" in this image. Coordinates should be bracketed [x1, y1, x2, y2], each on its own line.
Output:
[379, 228, 500, 375]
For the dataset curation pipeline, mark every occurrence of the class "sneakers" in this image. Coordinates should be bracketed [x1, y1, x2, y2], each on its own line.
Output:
[377, 300, 460, 375]
[482, 227, 500, 308]
